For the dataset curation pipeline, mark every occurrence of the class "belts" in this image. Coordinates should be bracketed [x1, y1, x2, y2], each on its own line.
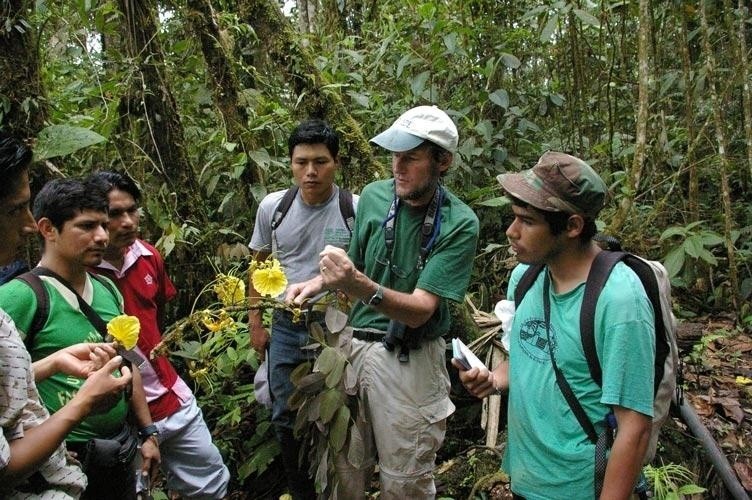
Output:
[68, 442, 86, 452]
[353, 330, 384, 342]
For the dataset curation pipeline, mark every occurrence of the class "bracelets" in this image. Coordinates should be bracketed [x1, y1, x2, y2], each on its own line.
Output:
[136, 423, 159, 441]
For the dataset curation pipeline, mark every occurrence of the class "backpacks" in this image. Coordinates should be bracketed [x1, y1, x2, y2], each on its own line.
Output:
[611, 253, 677, 466]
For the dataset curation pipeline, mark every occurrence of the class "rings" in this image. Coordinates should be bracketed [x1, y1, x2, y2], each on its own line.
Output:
[321, 266, 327, 271]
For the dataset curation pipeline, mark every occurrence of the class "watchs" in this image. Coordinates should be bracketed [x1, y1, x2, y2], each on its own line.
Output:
[361, 282, 384, 306]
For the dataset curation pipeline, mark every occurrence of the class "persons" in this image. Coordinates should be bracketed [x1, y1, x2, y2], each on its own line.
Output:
[245, 118, 364, 500]
[0, 174, 162, 500]
[1, 128, 133, 499]
[447, 146, 663, 500]
[88, 167, 232, 500]
[284, 102, 484, 500]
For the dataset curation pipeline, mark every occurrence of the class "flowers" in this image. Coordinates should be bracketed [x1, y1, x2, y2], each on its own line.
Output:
[103, 314, 141, 352]
[150, 244, 303, 396]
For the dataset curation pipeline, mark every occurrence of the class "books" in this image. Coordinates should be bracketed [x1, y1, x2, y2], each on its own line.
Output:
[452, 336, 501, 396]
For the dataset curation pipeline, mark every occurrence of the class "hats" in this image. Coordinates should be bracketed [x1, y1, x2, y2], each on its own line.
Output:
[369, 104, 459, 154]
[496, 152, 606, 221]
